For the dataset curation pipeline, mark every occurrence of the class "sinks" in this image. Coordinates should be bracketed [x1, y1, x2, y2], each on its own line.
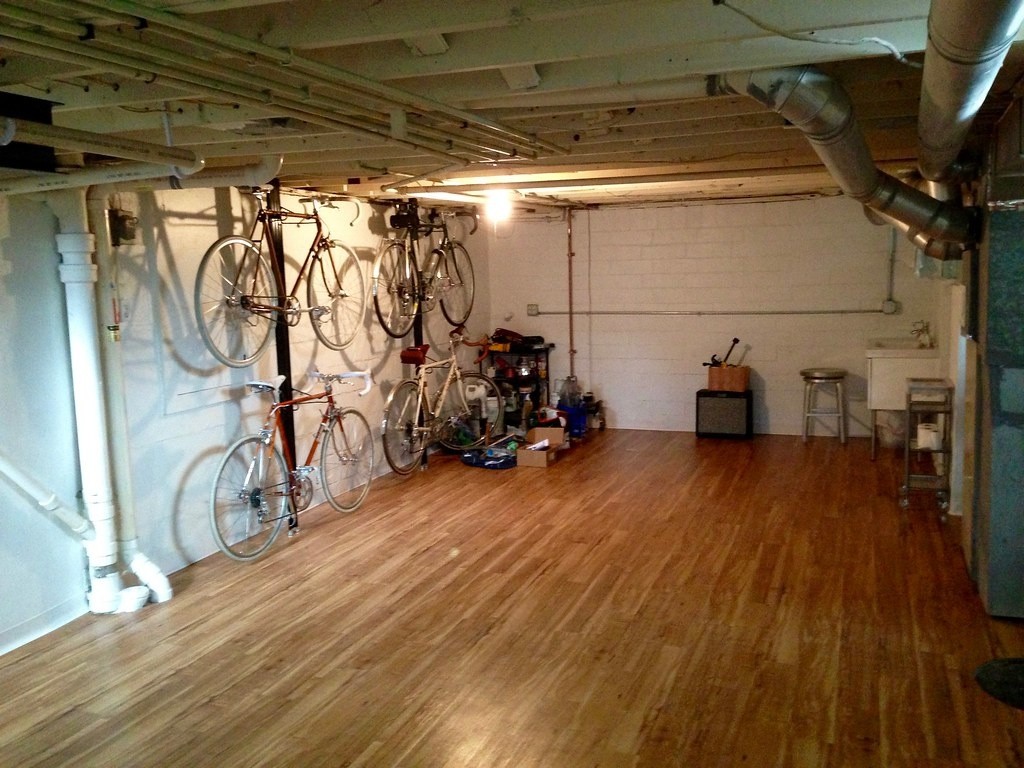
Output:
[865, 348, 941, 410]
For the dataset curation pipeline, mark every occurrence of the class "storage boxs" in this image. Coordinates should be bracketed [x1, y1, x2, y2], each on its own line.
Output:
[516, 427, 564, 467]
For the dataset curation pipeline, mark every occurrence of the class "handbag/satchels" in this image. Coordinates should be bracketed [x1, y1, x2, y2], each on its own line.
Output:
[556, 399, 587, 437]
[460, 451, 517, 469]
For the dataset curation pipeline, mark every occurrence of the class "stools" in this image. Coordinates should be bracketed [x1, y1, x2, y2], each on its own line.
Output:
[801, 367, 848, 443]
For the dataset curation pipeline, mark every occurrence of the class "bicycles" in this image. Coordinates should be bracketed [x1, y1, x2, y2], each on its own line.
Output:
[195, 181, 367, 368]
[208, 368, 374, 562]
[371, 203, 479, 339]
[379, 324, 504, 475]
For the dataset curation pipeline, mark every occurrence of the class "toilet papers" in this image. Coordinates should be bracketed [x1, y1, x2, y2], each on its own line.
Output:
[918, 423, 937, 427]
[930, 430, 940, 450]
[918, 427, 938, 448]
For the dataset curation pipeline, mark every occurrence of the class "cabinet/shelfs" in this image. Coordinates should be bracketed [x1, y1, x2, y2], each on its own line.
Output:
[481, 347, 550, 426]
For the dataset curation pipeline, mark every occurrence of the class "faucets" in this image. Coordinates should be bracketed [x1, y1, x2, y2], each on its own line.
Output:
[910, 320, 930, 347]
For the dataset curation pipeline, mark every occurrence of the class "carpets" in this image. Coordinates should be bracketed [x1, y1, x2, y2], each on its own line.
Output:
[977, 657, 1024, 710]
